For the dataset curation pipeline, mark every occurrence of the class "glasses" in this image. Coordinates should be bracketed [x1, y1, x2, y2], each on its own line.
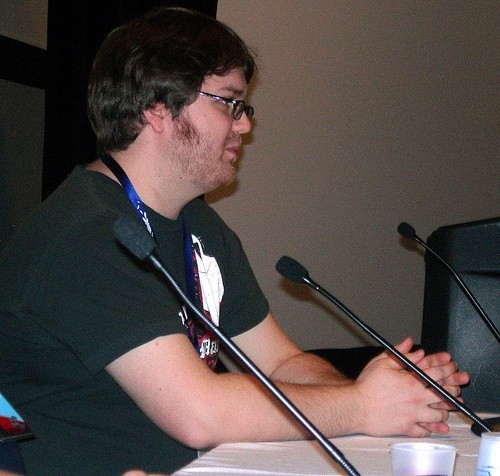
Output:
[194, 90, 254, 121]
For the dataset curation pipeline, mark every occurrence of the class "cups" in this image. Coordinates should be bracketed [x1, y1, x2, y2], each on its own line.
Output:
[389, 442, 458, 476]
[476, 431, 500, 476]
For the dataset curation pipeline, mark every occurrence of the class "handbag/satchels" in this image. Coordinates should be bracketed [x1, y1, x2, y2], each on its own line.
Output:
[420, 214, 500, 416]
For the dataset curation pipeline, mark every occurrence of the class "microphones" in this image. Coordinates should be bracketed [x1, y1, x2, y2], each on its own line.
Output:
[274, 256, 493, 433]
[395, 217, 500, 340]
[112, 216, 359, 476]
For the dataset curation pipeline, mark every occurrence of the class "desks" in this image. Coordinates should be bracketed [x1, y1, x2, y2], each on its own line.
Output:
[167, 401, 500, 476]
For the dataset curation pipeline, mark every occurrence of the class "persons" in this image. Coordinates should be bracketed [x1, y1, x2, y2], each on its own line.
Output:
[0, 8, 470, 476]
